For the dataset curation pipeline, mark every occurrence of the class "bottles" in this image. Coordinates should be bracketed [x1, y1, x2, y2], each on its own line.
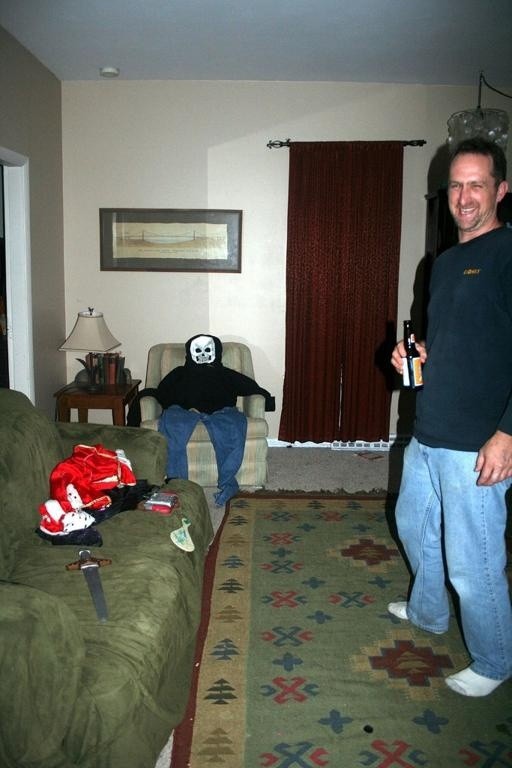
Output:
[397, 317, 426, 395]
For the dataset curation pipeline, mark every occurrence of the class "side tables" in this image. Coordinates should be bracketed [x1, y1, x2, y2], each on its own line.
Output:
[54, 378, 142, 426]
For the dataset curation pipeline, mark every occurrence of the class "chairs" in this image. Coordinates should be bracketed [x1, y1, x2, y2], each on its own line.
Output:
[141, 343, 268, 489]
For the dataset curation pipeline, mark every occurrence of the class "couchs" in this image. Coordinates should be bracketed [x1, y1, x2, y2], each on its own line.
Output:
[0, 385, 216, 768]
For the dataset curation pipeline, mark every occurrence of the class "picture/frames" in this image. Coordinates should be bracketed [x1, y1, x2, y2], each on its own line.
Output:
[99, 208, 242, 273]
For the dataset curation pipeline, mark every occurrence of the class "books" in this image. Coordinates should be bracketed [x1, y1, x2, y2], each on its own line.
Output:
[84, 352, 126, 386]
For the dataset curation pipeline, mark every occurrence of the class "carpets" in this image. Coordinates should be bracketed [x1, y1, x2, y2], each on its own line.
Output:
[169, 488, 512, 767]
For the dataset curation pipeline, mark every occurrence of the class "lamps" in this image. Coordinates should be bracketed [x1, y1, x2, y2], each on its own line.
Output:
[59, 307, 121, 392]
[448, 72, 512, 158]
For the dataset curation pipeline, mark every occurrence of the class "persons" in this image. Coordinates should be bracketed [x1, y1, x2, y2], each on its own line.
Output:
[385, 136, 512, 701]
